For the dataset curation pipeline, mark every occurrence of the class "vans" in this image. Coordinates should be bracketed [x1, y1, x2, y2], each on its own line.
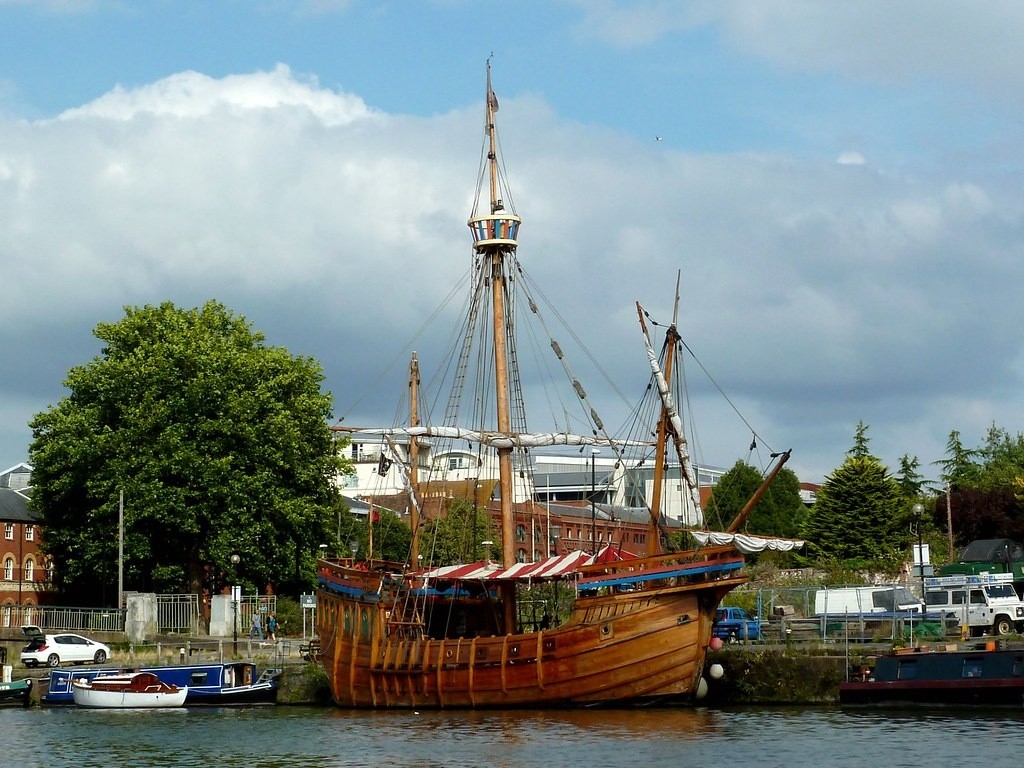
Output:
[814, 585, 923, 628]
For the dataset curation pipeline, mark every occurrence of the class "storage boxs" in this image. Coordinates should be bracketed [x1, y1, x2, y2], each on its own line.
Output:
[915, 647, 930, 652]
[939, 644, 958, 651]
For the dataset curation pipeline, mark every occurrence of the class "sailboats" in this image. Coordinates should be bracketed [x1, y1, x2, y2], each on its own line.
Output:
[296, 50, 792, 710]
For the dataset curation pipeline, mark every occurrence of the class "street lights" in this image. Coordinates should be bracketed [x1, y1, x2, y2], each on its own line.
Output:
[913, 504, 927, 622]
[231, 555, 240, 660]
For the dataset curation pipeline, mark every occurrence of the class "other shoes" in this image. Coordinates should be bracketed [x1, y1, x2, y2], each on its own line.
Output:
[275, 641, 279, 644]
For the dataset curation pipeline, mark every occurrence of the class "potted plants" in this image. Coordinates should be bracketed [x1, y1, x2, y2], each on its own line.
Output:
[891, 646, 915, 655]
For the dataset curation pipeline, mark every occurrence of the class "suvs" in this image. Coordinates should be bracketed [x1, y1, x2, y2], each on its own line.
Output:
[923, 573, 1024, 637]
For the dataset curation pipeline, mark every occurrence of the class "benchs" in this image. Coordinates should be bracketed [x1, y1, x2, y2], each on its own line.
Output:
[298, 639, 320, 659]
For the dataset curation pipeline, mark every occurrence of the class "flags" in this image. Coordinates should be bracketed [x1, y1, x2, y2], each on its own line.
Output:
[378, 452, 393, 477]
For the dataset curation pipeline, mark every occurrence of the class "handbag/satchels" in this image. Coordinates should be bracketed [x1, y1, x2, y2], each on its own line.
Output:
[263, 631, 269, 639]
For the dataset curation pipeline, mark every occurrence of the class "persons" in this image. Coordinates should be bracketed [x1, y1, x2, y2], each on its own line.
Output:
[250, 611, 264, 644]
[265, 611, 281, 644]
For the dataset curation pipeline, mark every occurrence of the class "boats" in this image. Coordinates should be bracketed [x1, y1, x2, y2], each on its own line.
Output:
[838, 640, 1024, 707]
[40, 663, 282, 706]
[0, 679, 33, 708]
[63, 671, 188, 710]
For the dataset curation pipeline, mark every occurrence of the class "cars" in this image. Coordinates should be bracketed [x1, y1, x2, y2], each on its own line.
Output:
[20, 625, 111, 669]
[711, 606, 759, 645]
[939, 538, 1024, 601]
